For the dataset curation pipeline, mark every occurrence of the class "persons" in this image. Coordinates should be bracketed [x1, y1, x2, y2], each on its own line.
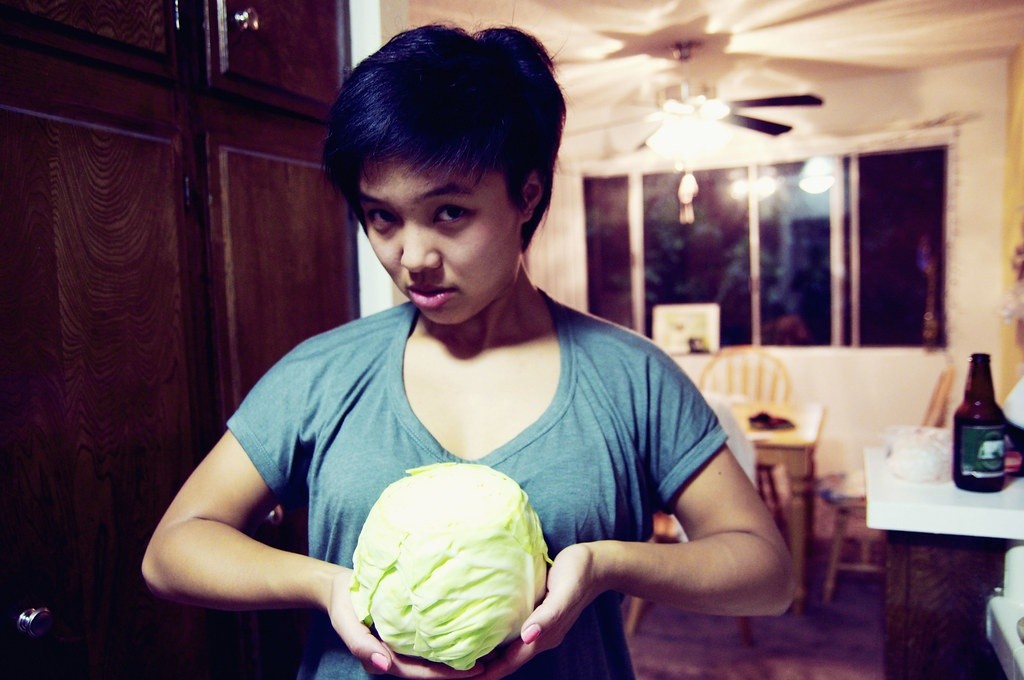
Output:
[141, 22, 796, 680]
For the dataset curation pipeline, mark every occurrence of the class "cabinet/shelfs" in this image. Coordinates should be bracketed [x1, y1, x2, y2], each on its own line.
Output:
[0, 0, 361, 679]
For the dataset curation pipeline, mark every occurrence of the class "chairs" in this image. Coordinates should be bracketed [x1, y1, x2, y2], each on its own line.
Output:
[617, 393, 765, 651]
[701, 344, 796, 552]
[819, 367, 957, 612]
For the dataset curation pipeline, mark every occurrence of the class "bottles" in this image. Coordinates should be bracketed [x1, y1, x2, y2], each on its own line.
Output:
[953, 352, 1008, 493]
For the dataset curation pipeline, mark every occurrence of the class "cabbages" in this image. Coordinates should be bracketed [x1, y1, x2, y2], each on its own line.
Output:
[348, 462, 555, 672]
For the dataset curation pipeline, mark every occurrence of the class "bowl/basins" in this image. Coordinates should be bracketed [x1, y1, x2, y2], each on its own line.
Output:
[885, 427, 948, 483]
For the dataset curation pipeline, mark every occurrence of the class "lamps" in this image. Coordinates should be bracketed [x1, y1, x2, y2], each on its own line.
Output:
[796, 158, 837, 194]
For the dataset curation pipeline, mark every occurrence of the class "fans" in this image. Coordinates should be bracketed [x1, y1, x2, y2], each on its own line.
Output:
[617, 43, 823, 153]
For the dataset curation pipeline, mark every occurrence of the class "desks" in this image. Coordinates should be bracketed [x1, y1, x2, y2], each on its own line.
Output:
[863, 446, 1024, 680]
[713, 399, 828, 618]
